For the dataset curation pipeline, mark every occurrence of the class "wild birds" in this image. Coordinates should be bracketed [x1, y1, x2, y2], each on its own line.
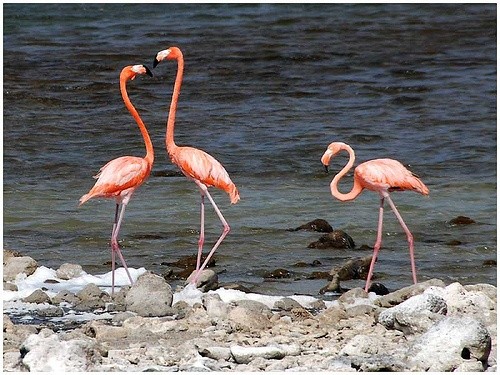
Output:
[151, 45, 242, 271]
[321, 140, 432, 294]
[76, 63, 155, 301]
